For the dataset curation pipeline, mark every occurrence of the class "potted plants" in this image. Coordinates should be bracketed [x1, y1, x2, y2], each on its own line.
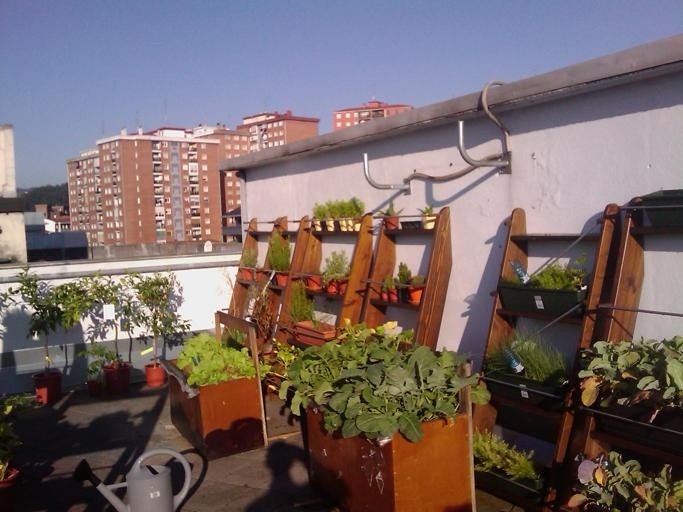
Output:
[121, 266, 191, 388]
[76, 341, 117, 397]
[0, 391, 38, 511]
[578, 335, 683, 446]
[487, 324, 571, 410]
[7, 264, 91, 404]
[234, 196, 438, 307]
[498, 261, 588, 316]
[473, 425, 548, 509]
[159, 331, 264, 463]
[291, 299, 336, 345]
[71, 268, 136, 392]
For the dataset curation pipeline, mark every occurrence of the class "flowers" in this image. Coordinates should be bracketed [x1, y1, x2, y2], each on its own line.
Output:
[278, 316, 492, 512]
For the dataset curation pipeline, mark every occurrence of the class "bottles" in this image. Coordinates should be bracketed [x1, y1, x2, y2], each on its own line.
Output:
[508, 354, 524, 375]
[515, 267, 529, 284]
